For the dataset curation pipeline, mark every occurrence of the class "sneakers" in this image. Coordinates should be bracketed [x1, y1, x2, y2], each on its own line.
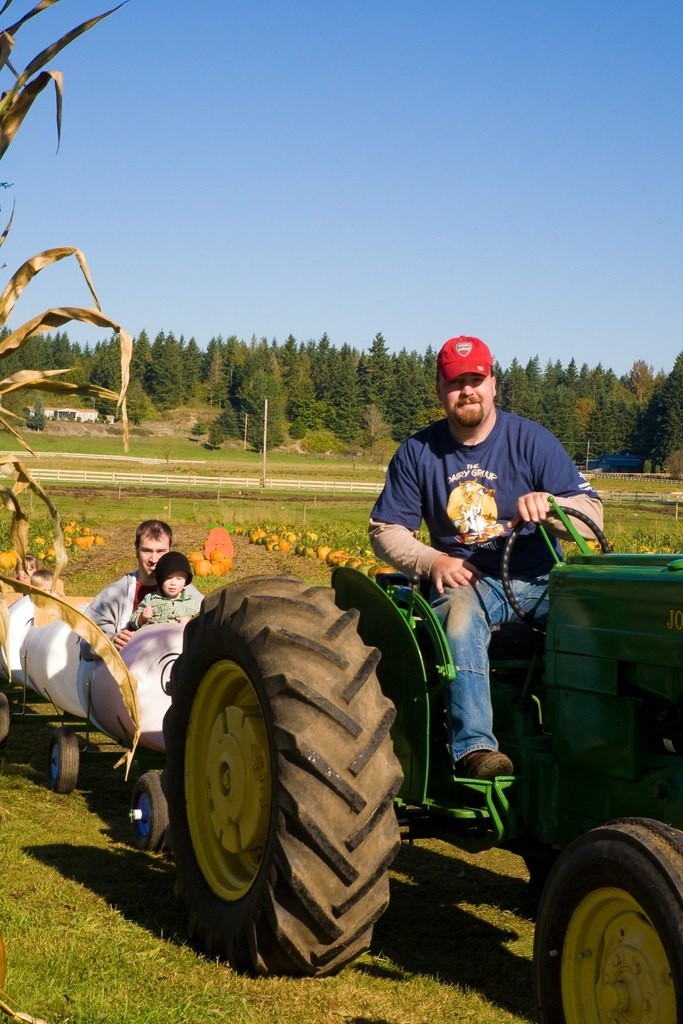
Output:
[456, 749, 513, 780]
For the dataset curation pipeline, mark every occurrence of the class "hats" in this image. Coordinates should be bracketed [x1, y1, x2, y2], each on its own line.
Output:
[437, 335, 493, 384]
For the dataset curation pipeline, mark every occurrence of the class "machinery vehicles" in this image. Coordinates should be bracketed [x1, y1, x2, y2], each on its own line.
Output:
[159, 504, 682, 1024]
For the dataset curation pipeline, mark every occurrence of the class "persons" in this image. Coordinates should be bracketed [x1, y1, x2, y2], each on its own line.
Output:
[368, 335, 603, 781]
[31, 571, 55, 596]
[79, 520, 205, 661]
[14, 552, 38, 586]
[132, 551, 202, 627]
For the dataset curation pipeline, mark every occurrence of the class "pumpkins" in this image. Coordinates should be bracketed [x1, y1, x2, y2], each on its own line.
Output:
[189, 540, 233, 577]
[0, 520, 106, 569]
[235, 524, 397, 582]
